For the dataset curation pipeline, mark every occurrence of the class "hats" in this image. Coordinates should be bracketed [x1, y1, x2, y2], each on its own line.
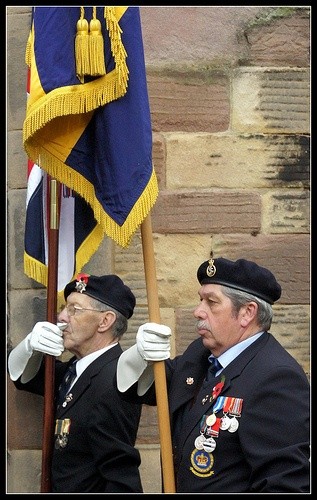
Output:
[196, 257, 281, 305]
[64, 273, 136, 319]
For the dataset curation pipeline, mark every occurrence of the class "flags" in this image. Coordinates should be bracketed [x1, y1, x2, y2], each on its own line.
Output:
[22, 5, 159, 288]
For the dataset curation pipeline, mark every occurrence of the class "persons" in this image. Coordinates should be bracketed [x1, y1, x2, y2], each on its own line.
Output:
[116, 256, 311, 495]
[8, 274, 143, 493]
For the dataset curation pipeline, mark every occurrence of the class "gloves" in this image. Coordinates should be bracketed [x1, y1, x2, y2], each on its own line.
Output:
[30, 321, 64, 357]
[136, 323, 172, 361]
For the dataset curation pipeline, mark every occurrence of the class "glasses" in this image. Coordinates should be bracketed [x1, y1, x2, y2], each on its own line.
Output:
[60, 304, 119, 323]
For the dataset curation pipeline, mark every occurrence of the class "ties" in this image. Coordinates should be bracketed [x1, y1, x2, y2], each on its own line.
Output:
[204, 357, 223, 384]
[58, 361, 77, 406]
